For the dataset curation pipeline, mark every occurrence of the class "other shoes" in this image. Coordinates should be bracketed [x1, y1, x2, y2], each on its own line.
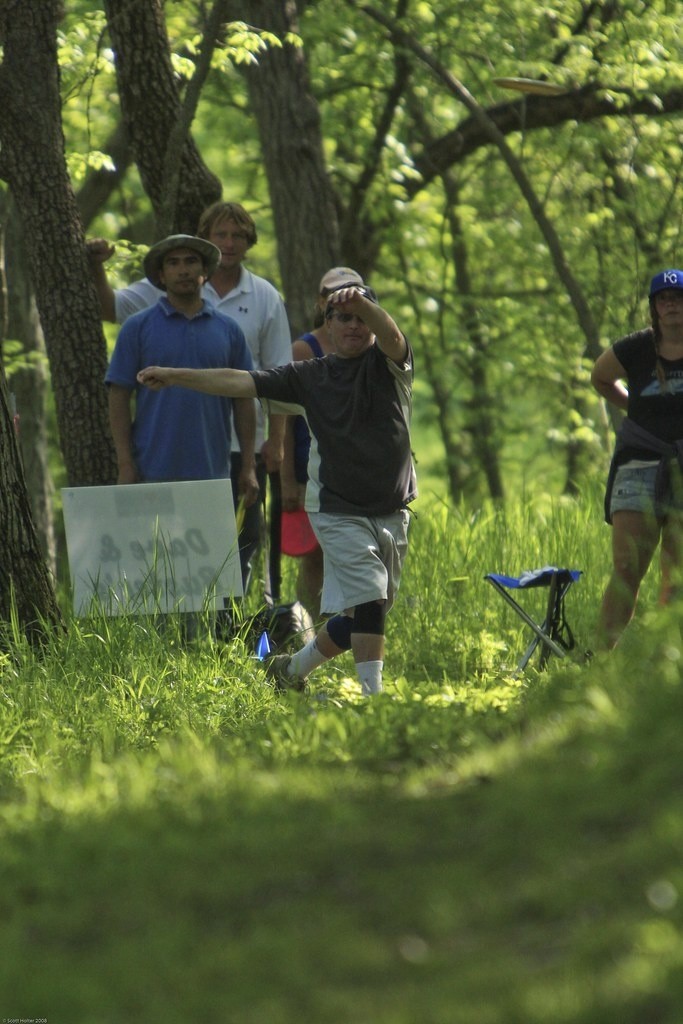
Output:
[579, 650, 606, 671]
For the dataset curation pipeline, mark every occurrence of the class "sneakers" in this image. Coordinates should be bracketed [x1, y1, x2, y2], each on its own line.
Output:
[265, 654, 311, 705]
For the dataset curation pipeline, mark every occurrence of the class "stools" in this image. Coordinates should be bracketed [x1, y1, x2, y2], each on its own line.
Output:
[485, 565, 584, 681]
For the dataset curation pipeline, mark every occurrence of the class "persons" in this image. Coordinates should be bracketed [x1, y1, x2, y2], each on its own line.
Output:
[137, 286, 419, 700]
[591, 271, 683, 654]
[101, 232, 263, 508]
[278, 266, 365, 629]
[84, 202, 292, 644]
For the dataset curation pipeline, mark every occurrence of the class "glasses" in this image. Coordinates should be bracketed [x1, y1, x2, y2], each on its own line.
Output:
[329, 312, 364, 323]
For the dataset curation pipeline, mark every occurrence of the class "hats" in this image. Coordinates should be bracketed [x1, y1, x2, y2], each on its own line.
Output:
[326, 281, 377, 314]
[319, 267, 365, 296]
[144, 234, 222, 291]
[649, 269, 683, 299]
